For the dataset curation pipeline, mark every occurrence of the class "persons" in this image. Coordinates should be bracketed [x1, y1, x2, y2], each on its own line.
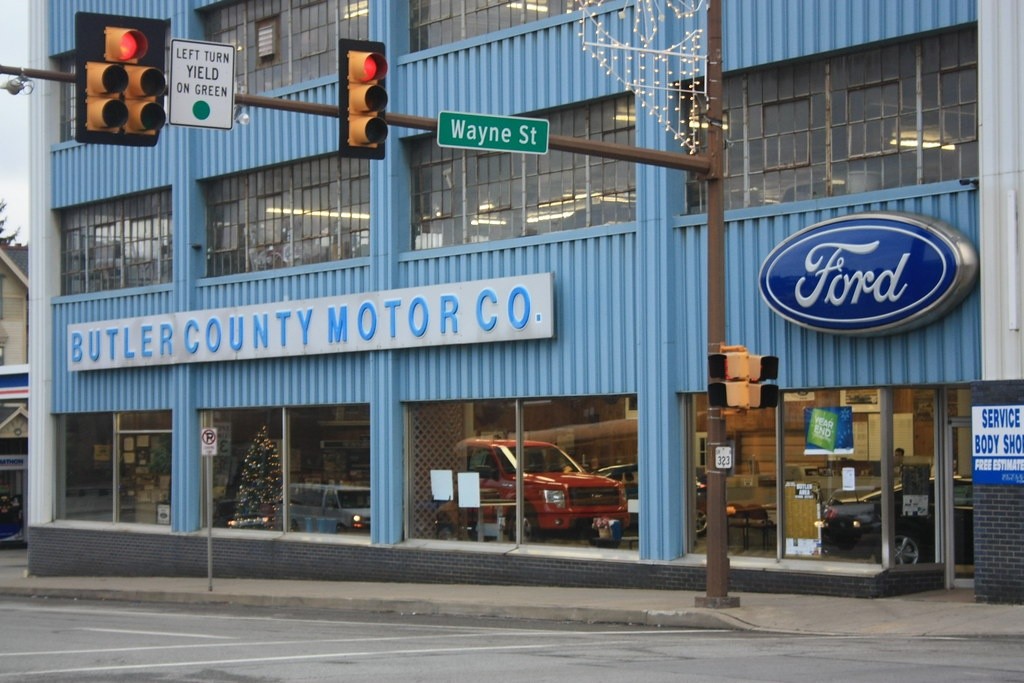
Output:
[895, 448, 904, 456]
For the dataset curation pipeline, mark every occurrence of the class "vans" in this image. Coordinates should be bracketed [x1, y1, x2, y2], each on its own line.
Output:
[288, 482, 370, 536]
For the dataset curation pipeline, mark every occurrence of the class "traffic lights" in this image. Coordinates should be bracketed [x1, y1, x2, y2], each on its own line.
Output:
[74, 11, 170, 147]
[337, 36, 388, 161]
[710, 345, 751, 409]
[749, 356, 780, 409]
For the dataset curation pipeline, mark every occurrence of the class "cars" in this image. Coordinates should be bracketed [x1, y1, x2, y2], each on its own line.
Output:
[820, 474, 973, 569]
[455, 436, 708, 549]
